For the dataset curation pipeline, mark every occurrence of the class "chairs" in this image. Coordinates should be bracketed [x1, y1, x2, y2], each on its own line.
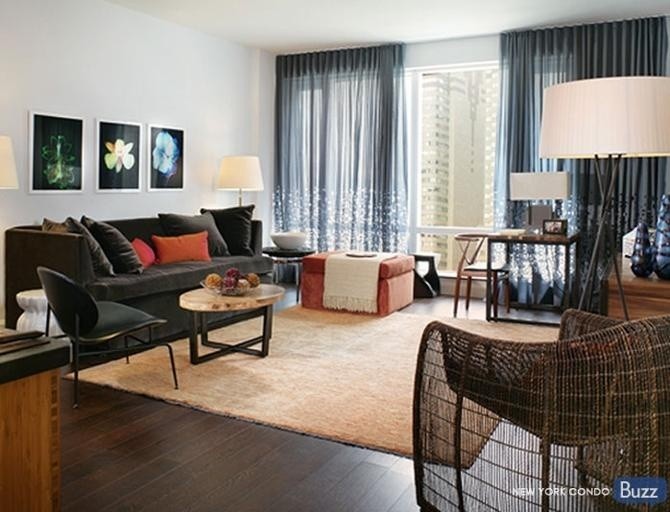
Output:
[417, 312, 670, 510]
[450, 232, 513, 324]
[35, 264, 180, 410]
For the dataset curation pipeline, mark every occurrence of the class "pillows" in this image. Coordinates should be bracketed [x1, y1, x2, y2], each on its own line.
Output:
[199, 204, 258, 259]
[151, 229, 213, 266]
[42, 214, 119, 280]
[156, 210, 234, 258]
[127, 235, 157, 271]
[79, 213, 145, 276]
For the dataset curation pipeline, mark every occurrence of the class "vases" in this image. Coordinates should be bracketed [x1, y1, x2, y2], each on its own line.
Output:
[627, 222, 654, 279]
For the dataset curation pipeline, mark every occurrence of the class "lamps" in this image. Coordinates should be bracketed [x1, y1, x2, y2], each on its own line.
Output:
[537, 75, 669, 322]
[0, 132, 22, 190]
[505, 169, 573, 238]
[214, 154, 266, 209]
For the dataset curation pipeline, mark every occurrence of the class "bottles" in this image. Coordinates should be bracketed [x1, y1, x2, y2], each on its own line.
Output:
[631, 221, 654, 278]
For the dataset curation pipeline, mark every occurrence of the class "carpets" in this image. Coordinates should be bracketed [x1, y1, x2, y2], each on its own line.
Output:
[59, 304, 558, 471]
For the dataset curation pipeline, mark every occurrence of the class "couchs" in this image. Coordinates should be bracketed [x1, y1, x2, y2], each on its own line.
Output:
[8, 218, 277, 358]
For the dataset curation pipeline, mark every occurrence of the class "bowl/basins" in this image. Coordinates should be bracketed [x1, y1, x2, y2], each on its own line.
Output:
[269, 232, 308, 250]
[199, 280, 258, 297]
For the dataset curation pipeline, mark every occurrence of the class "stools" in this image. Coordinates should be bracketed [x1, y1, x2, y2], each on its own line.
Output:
[300, 248, 417, 319]
[413, 252, 441, 298]
[15, 287, 76, 364]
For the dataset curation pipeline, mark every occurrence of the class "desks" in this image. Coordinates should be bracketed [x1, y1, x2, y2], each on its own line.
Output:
[1, 324, 76, 512]
[261, 245, 318, 304]
[597, 248, 670, 324]
[485, 228, 582, 330]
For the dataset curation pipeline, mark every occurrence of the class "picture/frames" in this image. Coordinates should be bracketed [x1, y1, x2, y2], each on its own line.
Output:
[543, 219, 568, 235]
[146, 122, 189, 193]
[26, 108, 87, 195]
[94, 116, 143, 194]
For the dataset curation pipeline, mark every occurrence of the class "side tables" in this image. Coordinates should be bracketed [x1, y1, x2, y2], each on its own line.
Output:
[177, 281, 287, 366]
